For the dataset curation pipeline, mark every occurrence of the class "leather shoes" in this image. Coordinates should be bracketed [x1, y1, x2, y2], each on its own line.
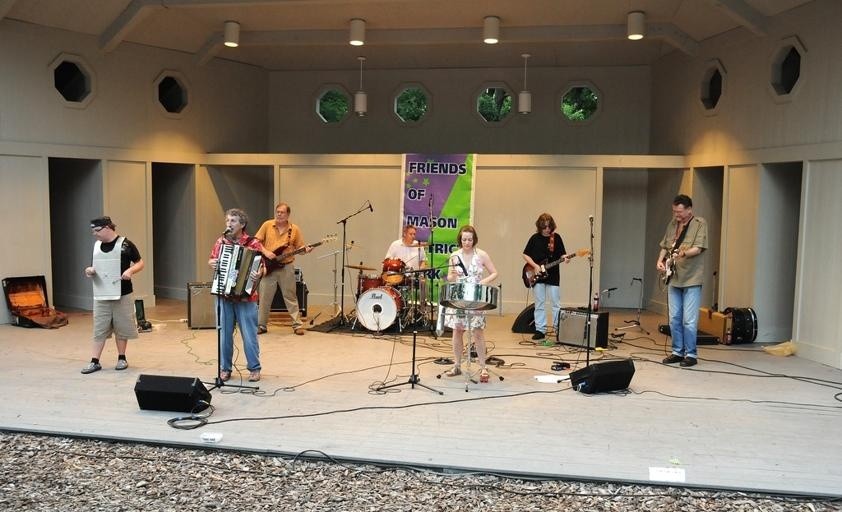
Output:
[680, 357, 697, 366]
[663, 354, 683, 364]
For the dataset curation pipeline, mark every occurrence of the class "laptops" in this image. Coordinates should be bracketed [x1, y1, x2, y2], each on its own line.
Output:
[135, 300, 151, 328]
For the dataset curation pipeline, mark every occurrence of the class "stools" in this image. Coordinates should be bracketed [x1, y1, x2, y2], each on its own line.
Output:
[396, 285, 412, 307]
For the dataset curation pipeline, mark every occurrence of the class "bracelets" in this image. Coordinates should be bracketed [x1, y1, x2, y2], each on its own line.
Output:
[681, 251, 686, 257]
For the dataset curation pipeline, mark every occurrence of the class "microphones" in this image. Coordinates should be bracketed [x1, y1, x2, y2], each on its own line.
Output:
[589, 215, 594, 238]
[633, 278, 642, 281]
[367, 200, 373, 212]
[222, 227, 234, 235]
[429, 194, 432, 206]
[350, 241, 354, 249]
[457, 256, 468, 277]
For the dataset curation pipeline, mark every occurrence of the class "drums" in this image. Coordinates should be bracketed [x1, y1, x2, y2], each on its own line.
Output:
[356, 275, 381, 293]
[356, 285, 406, 332]
[439, 283, 498, 310]
[404, 267, 416, 288]
[380, 257, 407, 283]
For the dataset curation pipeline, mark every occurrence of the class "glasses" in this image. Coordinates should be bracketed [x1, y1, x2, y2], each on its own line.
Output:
[93, 228, 103, 232]
[543, 225, 551, 228]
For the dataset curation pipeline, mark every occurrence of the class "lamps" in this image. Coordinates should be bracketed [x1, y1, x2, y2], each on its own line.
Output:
[353, 56, 368, 116]
[626, 9, 646, 40]
[349, 18, 367, 46]
[518, 50, 533, 115]
[223, 21, 241, 48]
[482, 15, 501, 45]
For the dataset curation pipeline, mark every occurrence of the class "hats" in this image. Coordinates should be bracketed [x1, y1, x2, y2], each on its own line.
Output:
[90, 216, 111, 227]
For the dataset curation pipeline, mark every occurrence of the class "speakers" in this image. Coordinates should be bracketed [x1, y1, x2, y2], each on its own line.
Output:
[186, 281, 218, 329]
[270, 282, 309, 317]
[568, 360, 635, 395]
[134, 373, 211, 413]
[557, 307, 609, 349]
[512, 303, 536, 333]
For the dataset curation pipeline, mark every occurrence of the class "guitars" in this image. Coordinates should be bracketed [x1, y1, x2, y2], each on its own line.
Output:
[264, 232, 337, 275]
[522, 249, 591, 287]
[660, 248, 680, 285]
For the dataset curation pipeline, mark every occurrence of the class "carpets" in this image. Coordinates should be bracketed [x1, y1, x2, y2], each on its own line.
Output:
[307, 316, 453, 339]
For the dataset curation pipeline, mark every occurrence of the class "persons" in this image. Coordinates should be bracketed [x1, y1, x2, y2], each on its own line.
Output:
[656, 194, 708, 370]
[205, 208, 267, 383]
[382, 224, 426, 314]
[252, 200, 312, 338]
[522, 213, 571, 342]
[81, 216, 145, 374]
[446, 224, 497, 383]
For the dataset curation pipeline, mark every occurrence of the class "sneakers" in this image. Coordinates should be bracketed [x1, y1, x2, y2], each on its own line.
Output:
[446, 367, 461, 376]
[81, 362, 101, 373]
[249, 372, 260, 382]
[296, 328, 304, 335]
[482, 369, 488, 377]
[219, 371, 229, 380]
[257, 328, 266, 334]
[116, 360, 127, 369]
[532, 332, 544, 339]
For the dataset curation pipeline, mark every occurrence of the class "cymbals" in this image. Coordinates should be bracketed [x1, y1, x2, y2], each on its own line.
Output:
[400, 241, 434, 247]
[344, 264, 378, 272]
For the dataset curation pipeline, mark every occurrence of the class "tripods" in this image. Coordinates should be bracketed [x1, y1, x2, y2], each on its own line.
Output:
[436, 315, 504, 392]
[375, 272, 444, 396]
[615, 288, 650, 338]
[308, 253, 350, 327]
